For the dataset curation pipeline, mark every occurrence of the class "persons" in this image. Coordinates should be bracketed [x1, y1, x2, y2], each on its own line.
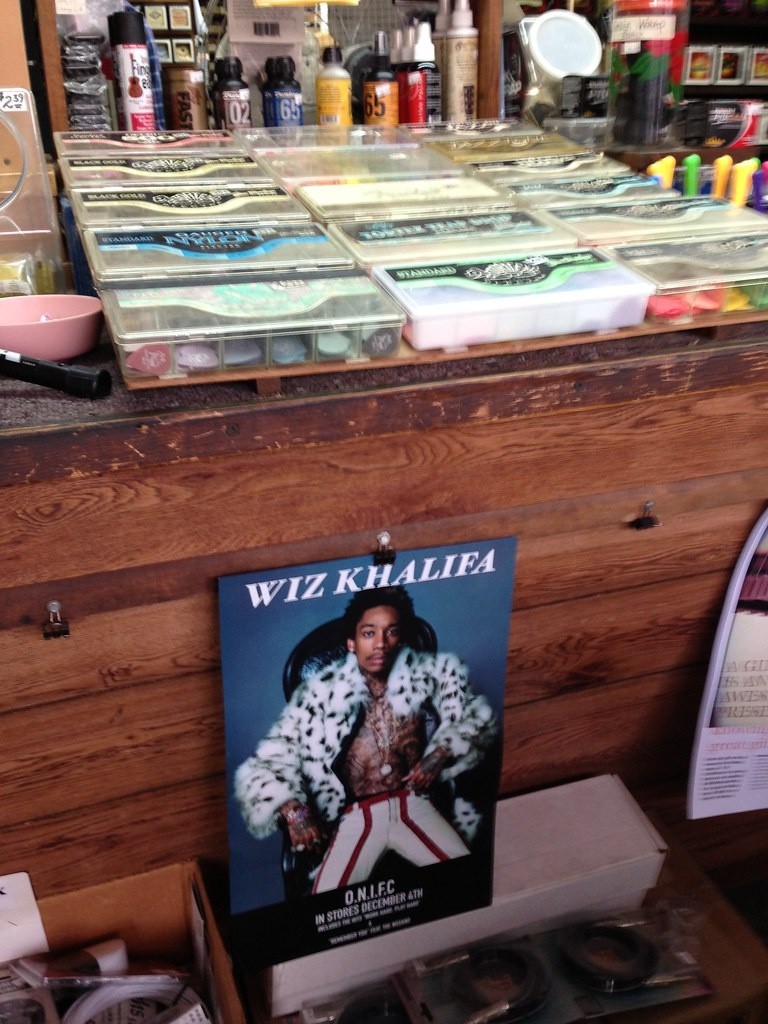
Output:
[235, 587, 497, 895]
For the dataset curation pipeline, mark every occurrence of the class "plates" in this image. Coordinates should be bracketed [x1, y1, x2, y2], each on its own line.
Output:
[530, 10, 602, 78]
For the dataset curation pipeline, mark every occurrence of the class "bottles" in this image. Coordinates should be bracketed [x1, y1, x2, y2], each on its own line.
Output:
[106, 0, 481, 128]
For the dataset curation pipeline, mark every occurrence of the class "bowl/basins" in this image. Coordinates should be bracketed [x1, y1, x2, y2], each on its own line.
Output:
[0, 294, 104, 362]
[544, 116, 616, 148]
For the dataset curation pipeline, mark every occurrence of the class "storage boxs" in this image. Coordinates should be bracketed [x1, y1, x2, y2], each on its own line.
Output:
[37, 856, 246, 1024]
[561, 73, 607, 119]
[49, 117, 768, 381]
[618, 837, 768, 1024]
[268, 769, 668, 1013]
[685, 101, 764, 149]
